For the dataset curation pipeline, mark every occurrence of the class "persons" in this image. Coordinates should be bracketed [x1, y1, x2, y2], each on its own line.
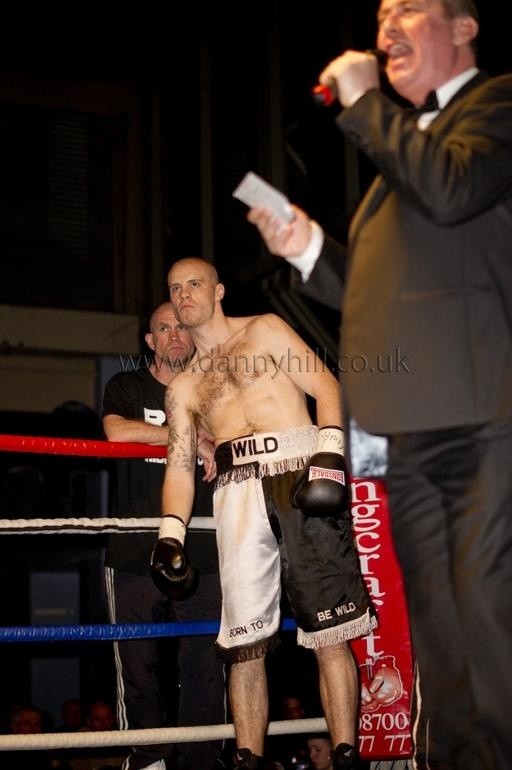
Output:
[248, 0, 512, 770]
[149, 258, 380, 770]
[265, 692, 334, 770]
[0, 692, 128, 770]
[104, 300, 230, 770]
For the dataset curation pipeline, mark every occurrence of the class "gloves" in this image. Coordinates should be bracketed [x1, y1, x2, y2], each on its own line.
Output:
[357, 657, 403, 713]
[291, 426, 349, 517]
[151, 515, 200, 602]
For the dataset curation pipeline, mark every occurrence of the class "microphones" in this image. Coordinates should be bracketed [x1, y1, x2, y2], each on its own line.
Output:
[311, 44, 390, 112]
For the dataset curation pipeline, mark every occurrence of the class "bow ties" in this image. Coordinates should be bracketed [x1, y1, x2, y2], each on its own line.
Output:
[404, 91, 438, 119]
